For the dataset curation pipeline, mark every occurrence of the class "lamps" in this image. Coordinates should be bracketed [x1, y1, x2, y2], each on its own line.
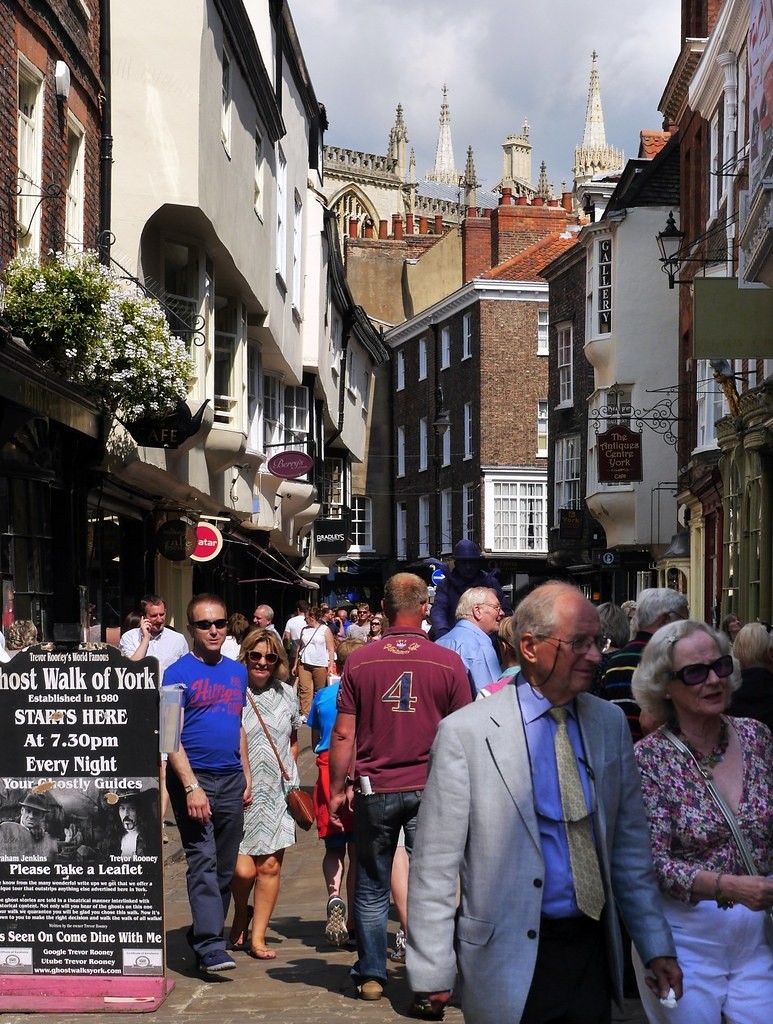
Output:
[655, 210, 687, 286]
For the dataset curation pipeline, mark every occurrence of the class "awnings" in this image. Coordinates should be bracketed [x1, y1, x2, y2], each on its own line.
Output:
[364, 554, 451, 575]
[0, 397, 323, 592]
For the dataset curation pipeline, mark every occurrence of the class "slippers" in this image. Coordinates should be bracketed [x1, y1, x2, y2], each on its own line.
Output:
[250, 941, 276, 960]
[229, 904, 255, 946]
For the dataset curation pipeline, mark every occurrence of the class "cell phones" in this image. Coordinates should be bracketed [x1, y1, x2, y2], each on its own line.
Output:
[140, 613, 147, 621]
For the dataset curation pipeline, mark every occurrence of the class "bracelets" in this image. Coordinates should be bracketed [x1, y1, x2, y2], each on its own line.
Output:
[713, 872, 734, 910]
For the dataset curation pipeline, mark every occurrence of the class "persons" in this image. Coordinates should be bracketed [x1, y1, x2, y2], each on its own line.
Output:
[404, 579, 685, 1024]
[0, 583, 439, 863]
[475, 615, 522, 702]
[630, 618, 773, 1024]
[326, 572, 475, 1018]
[583, 584, 773, 747]
[758, 59, 773, 169]
[227, 626, 304, 962]
[432, 585, 506, 696]
[304, 638, 368, 948]
[158, 591, 249, 972]
[379, 615, 462, 964]
[428, 538, 514, 666]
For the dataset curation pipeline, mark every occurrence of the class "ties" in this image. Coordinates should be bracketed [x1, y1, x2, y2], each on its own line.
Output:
[549, 706, 606, 920]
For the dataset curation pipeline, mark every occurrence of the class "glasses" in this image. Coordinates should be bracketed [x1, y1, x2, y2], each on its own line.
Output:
[248, 651, 279, 665]
[371, 622, 380, 626]
[534, 633, 612, 655]
[357, 611, 368, 614]
[192, 619, 228, 630]
[304, 616, 314, 621]
[669, 654, 734, 686]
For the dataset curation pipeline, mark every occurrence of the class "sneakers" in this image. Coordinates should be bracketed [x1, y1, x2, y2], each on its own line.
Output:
[324, 895, 349, 947]
[358, 979, 385, 1001]
[200, 947, 237, 972]
[390, 926, 407, 963]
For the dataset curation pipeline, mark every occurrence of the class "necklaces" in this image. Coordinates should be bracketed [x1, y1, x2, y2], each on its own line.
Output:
[669, 713, 731, 780]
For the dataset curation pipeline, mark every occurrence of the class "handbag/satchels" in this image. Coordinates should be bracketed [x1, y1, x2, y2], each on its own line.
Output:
[327, 672, 341, 687]
[292, 658, 301, 677]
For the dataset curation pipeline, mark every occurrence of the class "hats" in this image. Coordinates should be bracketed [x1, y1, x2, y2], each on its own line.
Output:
[18, 792, 52, 813]
[103, 783, 159, 805]
